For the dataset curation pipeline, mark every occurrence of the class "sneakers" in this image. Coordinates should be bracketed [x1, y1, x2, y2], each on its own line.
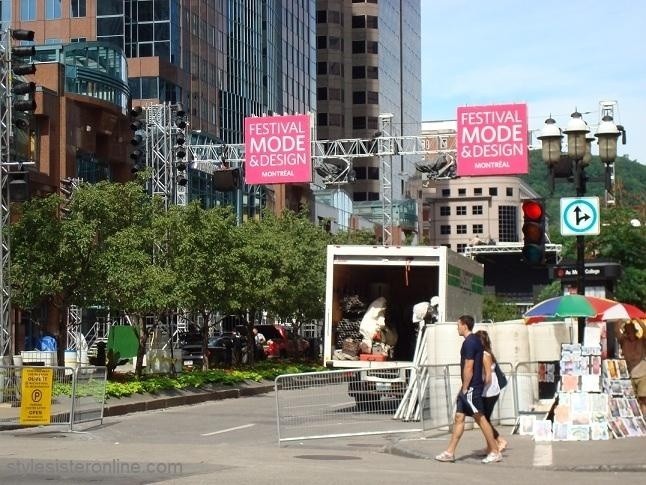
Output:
[479, 439, 507, 454]
[435, 452, 455, 462]
[482, 452, 503, 463]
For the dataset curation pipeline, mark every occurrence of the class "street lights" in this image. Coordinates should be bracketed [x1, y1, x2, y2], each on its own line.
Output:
[536, 107, 621, 345]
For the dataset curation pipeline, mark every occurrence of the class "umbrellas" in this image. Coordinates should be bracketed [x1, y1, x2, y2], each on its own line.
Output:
[521, 294, 646, 325]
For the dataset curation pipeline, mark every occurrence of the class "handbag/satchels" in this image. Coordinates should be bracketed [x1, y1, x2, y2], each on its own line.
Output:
[494, 365, 507, 388]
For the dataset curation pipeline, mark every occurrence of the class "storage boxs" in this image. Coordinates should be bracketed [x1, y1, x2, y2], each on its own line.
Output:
[359, 354, 386, 361]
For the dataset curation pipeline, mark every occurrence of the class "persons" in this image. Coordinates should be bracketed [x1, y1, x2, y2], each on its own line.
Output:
[614, 318, 646, 420]
[474, 329, 508, 454]
[253, 328, 266, 348]
[434, 314, 504, 464]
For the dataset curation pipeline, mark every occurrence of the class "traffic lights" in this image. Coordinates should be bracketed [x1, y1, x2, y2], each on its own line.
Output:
[522, 199, 545, 264]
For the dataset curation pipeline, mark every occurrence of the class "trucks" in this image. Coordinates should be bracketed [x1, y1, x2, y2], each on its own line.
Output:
[323, 244, 484, 411]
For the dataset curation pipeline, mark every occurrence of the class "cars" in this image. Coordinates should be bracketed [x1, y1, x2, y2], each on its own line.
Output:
[181, 324, 310, 372]
